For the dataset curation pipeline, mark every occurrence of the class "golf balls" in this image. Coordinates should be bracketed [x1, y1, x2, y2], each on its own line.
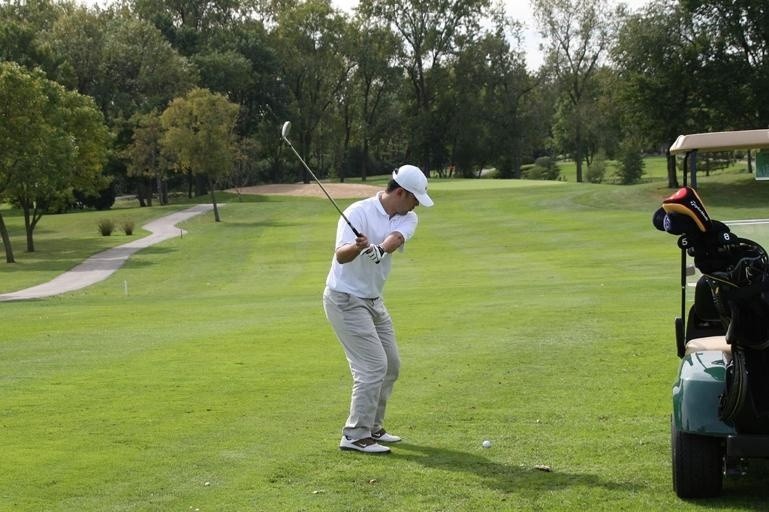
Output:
[483, 440, 491, 448]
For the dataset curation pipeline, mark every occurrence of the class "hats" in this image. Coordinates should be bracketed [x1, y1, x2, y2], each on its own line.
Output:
[393, 164, 434, 207]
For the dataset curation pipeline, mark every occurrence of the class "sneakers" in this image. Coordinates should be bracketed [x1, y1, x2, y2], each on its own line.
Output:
[339, 436, 391, 455]
[371, 428, 401, 442]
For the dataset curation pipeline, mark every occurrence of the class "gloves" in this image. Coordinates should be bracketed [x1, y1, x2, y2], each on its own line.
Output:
[360, 243, 387, 263]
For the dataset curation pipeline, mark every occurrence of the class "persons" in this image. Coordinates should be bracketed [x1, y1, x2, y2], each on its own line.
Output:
[322, 164, 433, 455]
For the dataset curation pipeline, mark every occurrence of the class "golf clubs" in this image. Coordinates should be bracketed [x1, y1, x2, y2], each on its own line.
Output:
[678, 229, 744, 271]
[281, 121, 380, 264]
